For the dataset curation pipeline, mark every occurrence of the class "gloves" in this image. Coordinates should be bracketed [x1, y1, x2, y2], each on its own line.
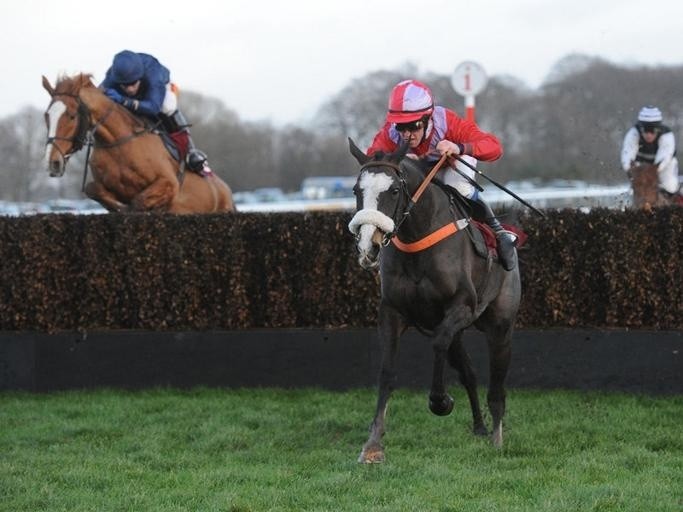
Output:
[104, 88, 125, 105]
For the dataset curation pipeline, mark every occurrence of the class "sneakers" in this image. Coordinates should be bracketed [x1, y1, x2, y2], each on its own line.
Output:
[496, 234, 516, 272]
[190, 153, 205, 167]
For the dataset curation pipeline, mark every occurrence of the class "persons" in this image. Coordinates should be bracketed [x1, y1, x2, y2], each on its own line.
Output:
[367, 78, 517, 272]
[620, 103, 681, 196]
[96, 49, 204, 167]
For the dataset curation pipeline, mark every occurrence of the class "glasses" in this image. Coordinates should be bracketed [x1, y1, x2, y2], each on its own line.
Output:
[395, 120, 420, 131]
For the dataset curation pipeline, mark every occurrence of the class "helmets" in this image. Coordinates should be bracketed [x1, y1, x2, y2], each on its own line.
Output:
[637, 105, 662, 128]
[110, 50, 144, 85]
[386, 80, 434, 124]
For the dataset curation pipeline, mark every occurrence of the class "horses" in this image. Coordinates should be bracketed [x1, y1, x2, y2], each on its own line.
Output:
[38, 70, 237, 215]
[624, 157, 681, 208]
[342, 130, 524, 465]
